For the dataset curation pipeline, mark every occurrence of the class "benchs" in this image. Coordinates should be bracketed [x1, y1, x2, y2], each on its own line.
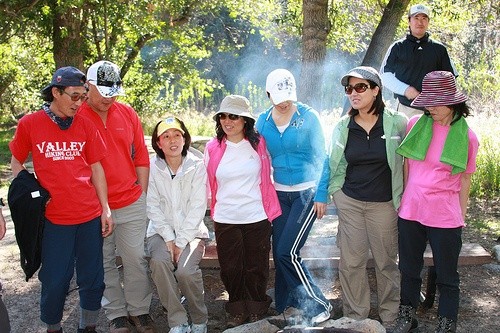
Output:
[146, 243, 492, 308]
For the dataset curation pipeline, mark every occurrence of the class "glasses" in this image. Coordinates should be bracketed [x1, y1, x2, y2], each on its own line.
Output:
[345, 83, 372, 95]
[60, 89, 89, 103]
[218, 113, 244, 120]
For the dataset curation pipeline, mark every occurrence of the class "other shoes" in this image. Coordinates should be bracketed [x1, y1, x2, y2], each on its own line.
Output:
[169, 319, 208, 333]
[390, 305, 419, 333]
[47, 327, 63, 333]
[78, 326, 98, 333]
[227, 312, 261, 328]
[433, 315, 457, 333]
[110, 314, 155, 333]
[382, 321, 393, 333]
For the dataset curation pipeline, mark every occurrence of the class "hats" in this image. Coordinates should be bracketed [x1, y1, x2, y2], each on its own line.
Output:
[212, 95, 256, 123]
[341, 66, 384, 89]
[86, 60, 126, 98]
[42, 66, 89, 97]
[266, 68, 297, 105]
[410, 71, 468, 107]
[408, 4, 430, 18]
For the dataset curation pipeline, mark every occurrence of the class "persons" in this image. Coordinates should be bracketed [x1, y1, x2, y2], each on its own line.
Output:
[70, 60, 156, 333]
[0, 195, 13, 333]
[252, 68, 337, 324]
[388, 70, 481, 333]
[376, 3, 459, 130]
[6, 65, 115, 333]
[204, 93, 284, 328]
[143, 116, 215, 333]
[329, 66, 411, 330]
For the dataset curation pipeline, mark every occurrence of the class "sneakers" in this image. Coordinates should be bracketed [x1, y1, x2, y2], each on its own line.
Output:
[310, 302, 333, 327]
